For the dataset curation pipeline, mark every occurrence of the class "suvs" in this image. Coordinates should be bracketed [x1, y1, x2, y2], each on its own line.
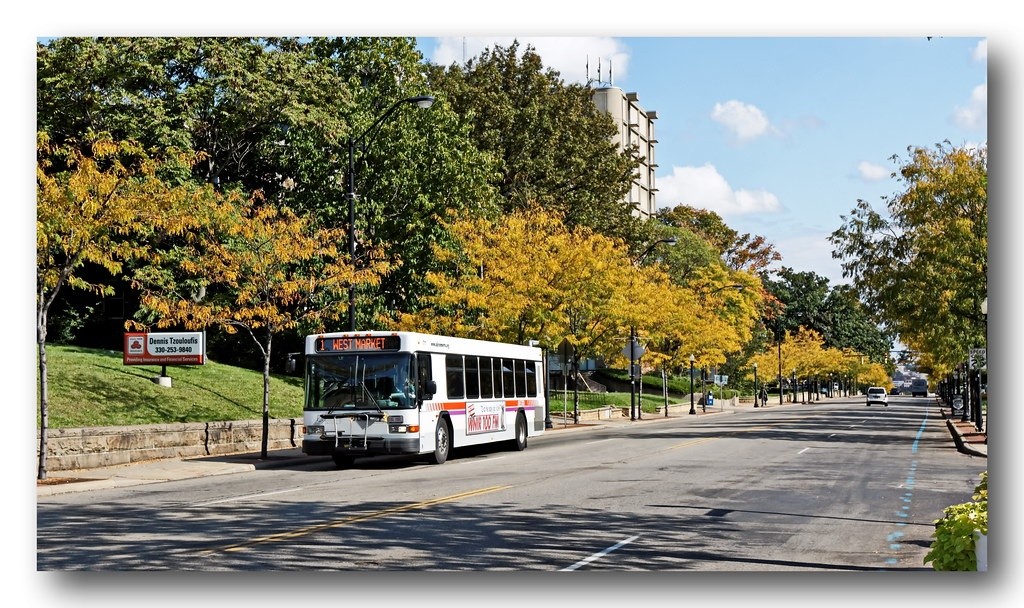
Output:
[866, 387, 888, 406]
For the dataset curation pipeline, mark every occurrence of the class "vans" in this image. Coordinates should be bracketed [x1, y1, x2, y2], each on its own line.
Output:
[911, 379, 928, 397]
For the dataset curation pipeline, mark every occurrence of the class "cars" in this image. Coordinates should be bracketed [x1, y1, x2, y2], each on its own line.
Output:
[890, 388, 900, 395]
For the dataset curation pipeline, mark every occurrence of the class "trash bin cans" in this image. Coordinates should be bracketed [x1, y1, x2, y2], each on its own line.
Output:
[707, 390, 712, 405]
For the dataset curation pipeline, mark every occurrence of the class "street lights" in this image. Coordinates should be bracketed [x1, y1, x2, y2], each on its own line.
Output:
[815, 371, 848, 400]
[981, 297, 988, 444]
[629, 236, 678, 419]
[792, 368, 798, 403]
[345, 94, 437, 327]
[700, 284, 743, 413]
[776, 312, 803, 405]
[753, 363, 759, 408]
[687, 353, 697, 415]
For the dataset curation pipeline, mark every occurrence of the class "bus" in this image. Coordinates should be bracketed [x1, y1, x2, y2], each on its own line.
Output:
[284, 330, 548, 466]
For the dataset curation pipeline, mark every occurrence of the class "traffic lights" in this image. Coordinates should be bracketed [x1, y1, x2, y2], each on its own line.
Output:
[884, 352, 888, 364]
[859, 356, 863, 366]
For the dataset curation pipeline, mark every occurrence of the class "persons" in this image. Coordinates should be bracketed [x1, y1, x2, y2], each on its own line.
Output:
[393, 371, 416, 394]
[758, 385, 768, 406]
